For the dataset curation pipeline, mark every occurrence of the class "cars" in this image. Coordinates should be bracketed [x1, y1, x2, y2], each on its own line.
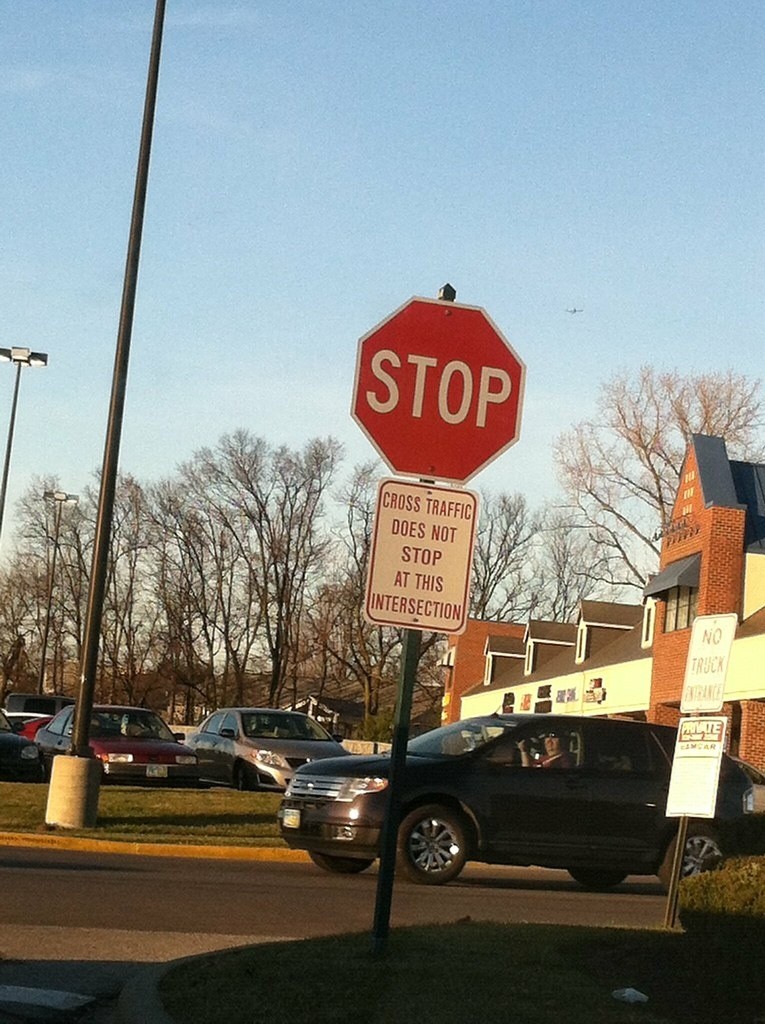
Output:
[275, 710, 758, 896]
[181, 706, 353, 795]
[0, 693, 122, 784]
[32, 702, 202, 789]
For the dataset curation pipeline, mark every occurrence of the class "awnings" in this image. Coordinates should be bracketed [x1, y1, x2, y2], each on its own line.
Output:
[643, 553, 701, 597]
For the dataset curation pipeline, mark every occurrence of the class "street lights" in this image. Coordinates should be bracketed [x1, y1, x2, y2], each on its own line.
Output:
[1, 345, 47, 532]
[36, 490, 80, 692]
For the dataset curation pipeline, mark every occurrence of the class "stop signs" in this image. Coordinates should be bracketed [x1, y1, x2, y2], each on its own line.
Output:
[348, 293, 527, 485]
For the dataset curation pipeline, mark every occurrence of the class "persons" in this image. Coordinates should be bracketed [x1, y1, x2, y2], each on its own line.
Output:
[516, 729, 571, 768]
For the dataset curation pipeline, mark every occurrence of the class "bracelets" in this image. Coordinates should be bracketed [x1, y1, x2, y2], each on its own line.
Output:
[521, 751, 529, 755]
